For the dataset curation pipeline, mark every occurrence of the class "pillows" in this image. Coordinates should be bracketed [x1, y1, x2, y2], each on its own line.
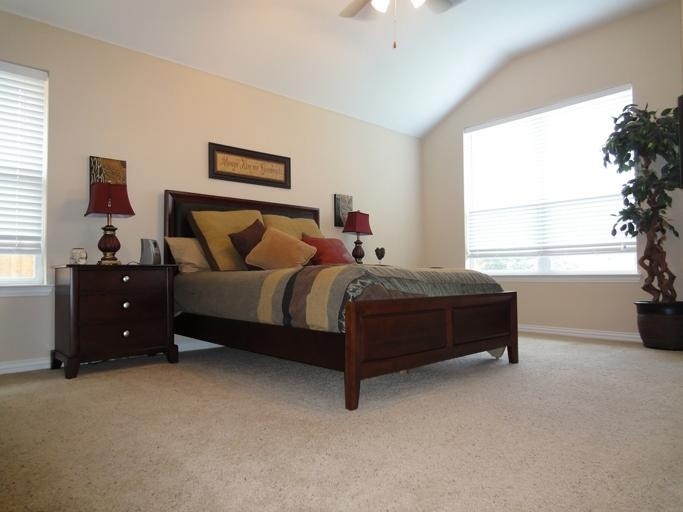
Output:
[163, 236, 211, 274]
[227, 218, 266, 271]
[187, 209, 267, 272]
[300, 231, 356, 266]
[243, 226, 316, 271]
[262, 214, 324, 241]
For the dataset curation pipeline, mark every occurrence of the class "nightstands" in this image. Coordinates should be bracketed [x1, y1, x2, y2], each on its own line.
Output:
[47, 263, 179, 380]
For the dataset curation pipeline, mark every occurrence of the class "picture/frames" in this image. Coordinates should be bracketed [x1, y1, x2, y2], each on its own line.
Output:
[206, 141, 292, 190]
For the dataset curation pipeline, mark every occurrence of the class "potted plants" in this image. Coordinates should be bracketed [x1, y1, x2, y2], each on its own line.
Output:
[601, 93, 682, 353]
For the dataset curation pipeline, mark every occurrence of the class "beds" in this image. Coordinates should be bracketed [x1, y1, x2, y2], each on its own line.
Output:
[164, 188, 520, 412]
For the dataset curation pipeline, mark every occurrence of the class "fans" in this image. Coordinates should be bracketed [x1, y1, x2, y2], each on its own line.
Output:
[336, 0, 453, 18]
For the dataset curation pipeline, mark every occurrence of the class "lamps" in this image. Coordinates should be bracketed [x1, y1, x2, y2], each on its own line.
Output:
[84, 155, 135, 265]
[341, 210, 374, 264]
[368, 0, 426, 14]
